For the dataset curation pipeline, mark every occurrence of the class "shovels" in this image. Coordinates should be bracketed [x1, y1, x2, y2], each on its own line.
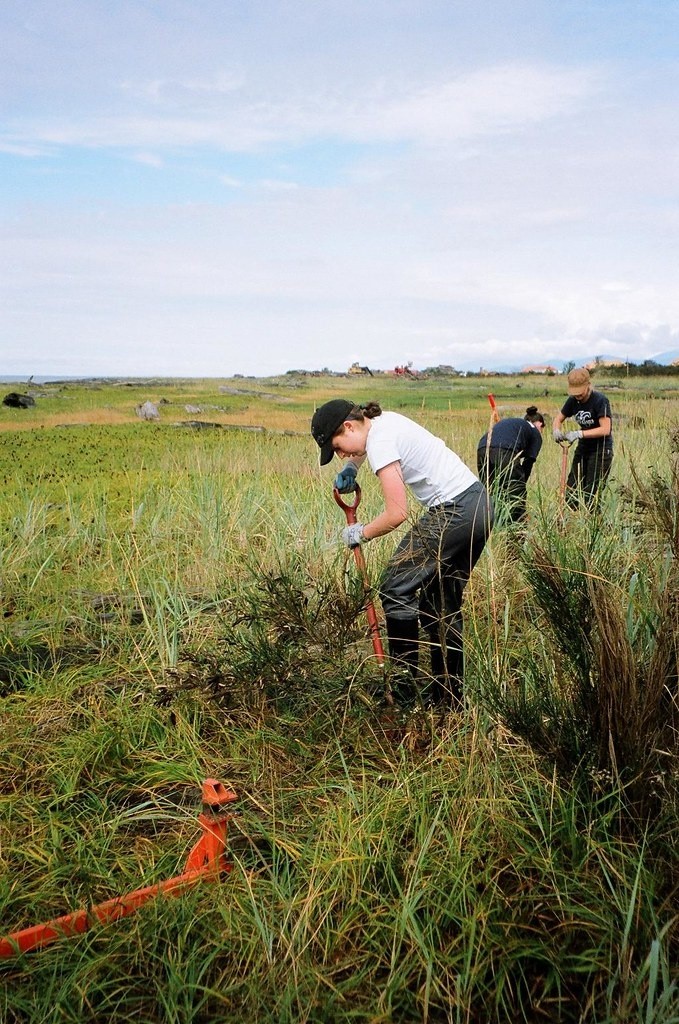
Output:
[330, 480, 419, 734]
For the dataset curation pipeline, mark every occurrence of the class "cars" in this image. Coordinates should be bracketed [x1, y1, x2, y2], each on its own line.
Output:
[3, 392, 36, 408]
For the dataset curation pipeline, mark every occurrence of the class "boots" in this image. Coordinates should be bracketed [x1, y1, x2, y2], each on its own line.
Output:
[370, 617, 419, 706]
[419, 629, 469, 713]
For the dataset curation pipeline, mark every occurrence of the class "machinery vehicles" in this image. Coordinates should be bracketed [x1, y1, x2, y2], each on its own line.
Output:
[348, 362, 374, 376]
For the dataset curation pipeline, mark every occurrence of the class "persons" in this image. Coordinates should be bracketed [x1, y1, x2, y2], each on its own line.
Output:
[477, 407, 545, 563]
[552, 367, 614, 514]
[311, 398, 494, 714]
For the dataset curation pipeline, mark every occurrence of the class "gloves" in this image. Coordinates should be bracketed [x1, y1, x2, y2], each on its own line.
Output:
[333, 461, 359, 494]
[553, 429, 563, 443]
[344, 522, 369, 549]
[563, 430, 583, 444]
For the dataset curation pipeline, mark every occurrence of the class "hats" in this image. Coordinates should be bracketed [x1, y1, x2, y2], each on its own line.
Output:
[567, 368, 590, 395]
[310, 398, 354, 466]
